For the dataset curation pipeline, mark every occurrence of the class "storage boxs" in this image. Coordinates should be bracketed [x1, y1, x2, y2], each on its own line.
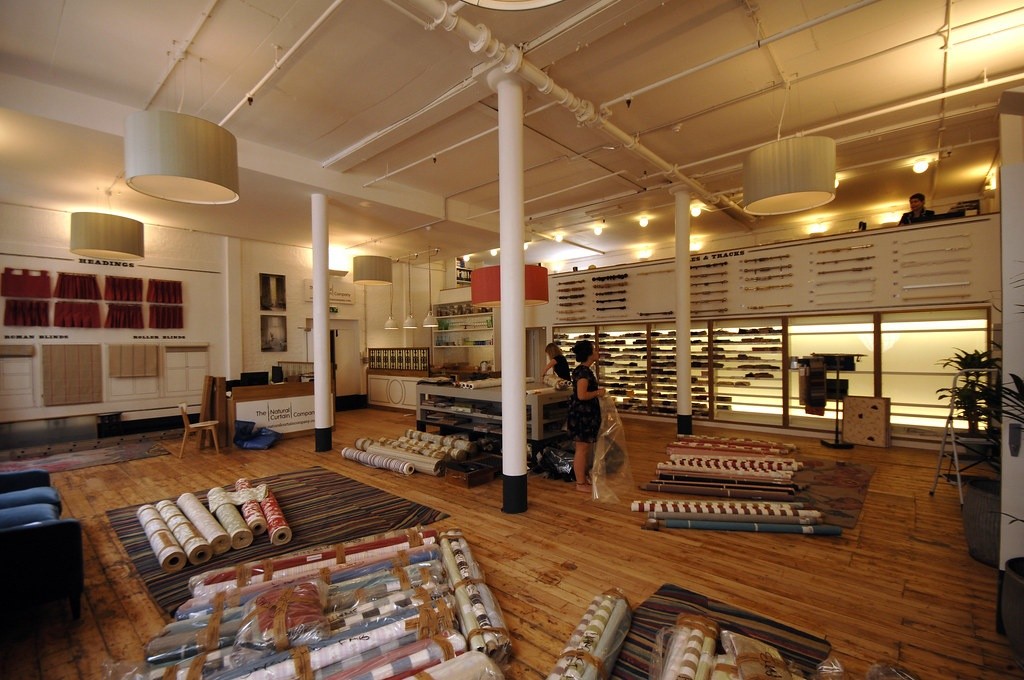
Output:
[446, 454, 503, 488]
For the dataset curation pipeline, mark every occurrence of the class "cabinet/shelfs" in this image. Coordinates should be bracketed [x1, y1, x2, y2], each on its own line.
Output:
[416, 380, 575, 470]
[430, 303, 494, 375]
[552, 308, 992, 439]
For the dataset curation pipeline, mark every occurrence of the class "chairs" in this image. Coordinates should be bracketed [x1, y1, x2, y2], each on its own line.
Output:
[179, 402, 219, 458]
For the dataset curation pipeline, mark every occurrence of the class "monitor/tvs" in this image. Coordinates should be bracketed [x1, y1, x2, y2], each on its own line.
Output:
[241, 372, 269, 387]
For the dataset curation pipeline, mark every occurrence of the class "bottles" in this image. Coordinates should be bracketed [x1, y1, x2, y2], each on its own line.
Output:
[436, 304, 494, 345]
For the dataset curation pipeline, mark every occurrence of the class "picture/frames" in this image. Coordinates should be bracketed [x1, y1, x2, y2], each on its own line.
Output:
[258, 272, 288, 352]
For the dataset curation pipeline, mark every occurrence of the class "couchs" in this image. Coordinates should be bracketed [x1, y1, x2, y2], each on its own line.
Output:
[0, 470, 82, 631]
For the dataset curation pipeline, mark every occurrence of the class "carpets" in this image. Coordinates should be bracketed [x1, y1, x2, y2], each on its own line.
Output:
[796, 452, 876, 527]
[610, 583, 830, 680]
[105, 465, 450, 615]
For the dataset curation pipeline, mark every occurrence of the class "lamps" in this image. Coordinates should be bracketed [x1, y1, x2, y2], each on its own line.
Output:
[353, 246, 440, 330]
[472, 265, 548, 307]
[68, 112, 239, 261]
[742, 134, 834, 218]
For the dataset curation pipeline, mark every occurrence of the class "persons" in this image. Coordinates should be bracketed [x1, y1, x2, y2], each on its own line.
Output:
[898, 193, 939, 225]
[541, 343, 571, 381]
[570, 340, 607, 493]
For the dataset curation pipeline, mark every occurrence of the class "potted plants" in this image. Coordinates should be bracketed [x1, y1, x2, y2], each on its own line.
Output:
[936, 340, 1002, 568]
[959, 373, 1024, 655]
